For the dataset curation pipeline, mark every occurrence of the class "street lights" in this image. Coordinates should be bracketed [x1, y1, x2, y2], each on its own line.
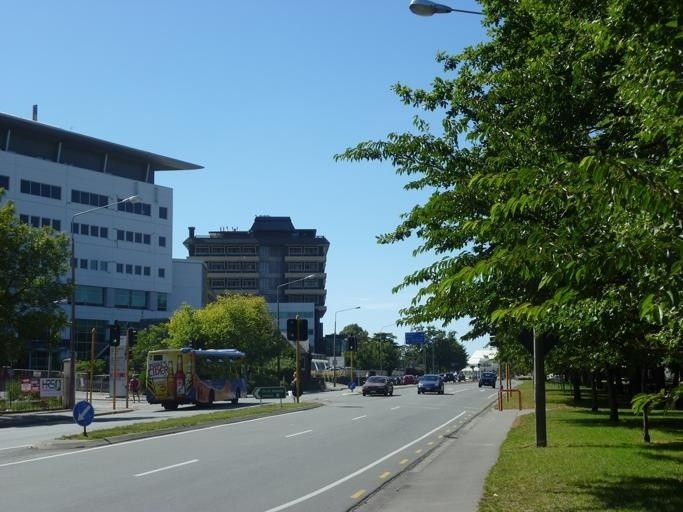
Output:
[68, 195, 143, 406]
[408, 0, 544, 450]
[332, 306, 360, 388]
[276, 274, 315, 388]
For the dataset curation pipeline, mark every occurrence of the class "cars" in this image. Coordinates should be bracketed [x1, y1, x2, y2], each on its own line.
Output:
[389, 374, 417, 385]
[417, 375, 443, 394]
[316, 368, 345, 379]
[361, 375, 393, 397]
[478, 372, 497, 388]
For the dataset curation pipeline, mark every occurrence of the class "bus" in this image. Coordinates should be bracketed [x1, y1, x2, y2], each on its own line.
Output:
[145, 347, 249, 411]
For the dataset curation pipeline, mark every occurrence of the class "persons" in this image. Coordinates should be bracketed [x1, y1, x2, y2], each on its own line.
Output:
[290, 371, 297, 403]
[129, 374, 141, 404]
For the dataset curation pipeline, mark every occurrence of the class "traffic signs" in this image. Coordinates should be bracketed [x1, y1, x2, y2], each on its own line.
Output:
[347, 382, 355, 390]
[251, 386, 286, 399]
[72, 401, 94, 427]
[404, 332, 424, 344]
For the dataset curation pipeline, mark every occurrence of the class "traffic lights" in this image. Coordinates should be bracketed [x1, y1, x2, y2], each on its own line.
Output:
[109, 325, 120, 346]
[127, 327, 137, 347]
